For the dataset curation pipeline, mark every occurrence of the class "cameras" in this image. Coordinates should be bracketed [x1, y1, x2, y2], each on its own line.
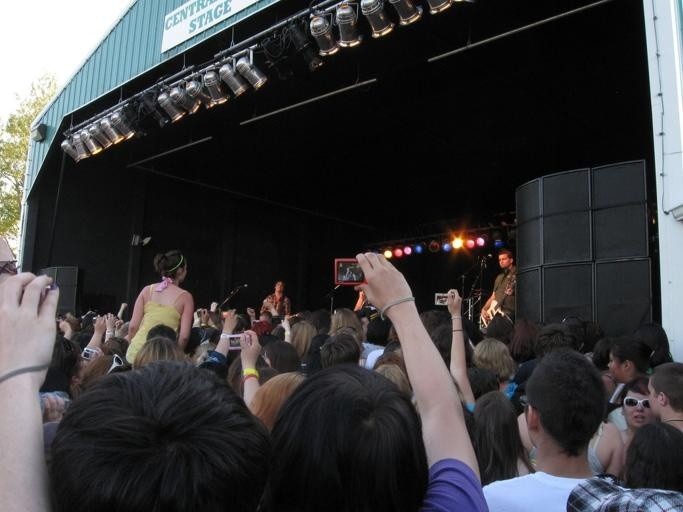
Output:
[92, 317, 97, 327]
[334, 258, 368, 285]
[434, 293, 454, 306]
[197, 310, 201, 318]
[81, 347, 97, 360]
[227, 334, 251, 350]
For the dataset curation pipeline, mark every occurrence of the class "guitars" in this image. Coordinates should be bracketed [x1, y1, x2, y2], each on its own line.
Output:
[479, 280, 516, 334]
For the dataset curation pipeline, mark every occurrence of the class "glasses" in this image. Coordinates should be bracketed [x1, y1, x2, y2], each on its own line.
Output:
[106, 354, 123, 374]
[624, 397, 650, 409]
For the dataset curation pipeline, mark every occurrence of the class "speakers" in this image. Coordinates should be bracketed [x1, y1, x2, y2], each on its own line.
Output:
[514, 159, 653, 341]
[39, 266, 79, 320]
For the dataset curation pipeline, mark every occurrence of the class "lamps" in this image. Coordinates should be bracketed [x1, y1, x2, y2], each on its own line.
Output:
[382, 233, 505, 260]
[307, 0, 456, 58]
[31, 124, 47, 142]
[59, 42, 268, 162]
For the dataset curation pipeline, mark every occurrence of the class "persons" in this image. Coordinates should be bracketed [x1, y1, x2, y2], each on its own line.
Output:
[1, 250, 683, 511]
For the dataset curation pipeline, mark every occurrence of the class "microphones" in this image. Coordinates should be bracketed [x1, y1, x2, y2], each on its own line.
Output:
[483, 253, 493, 260]
[235, 283, 248, 289]
